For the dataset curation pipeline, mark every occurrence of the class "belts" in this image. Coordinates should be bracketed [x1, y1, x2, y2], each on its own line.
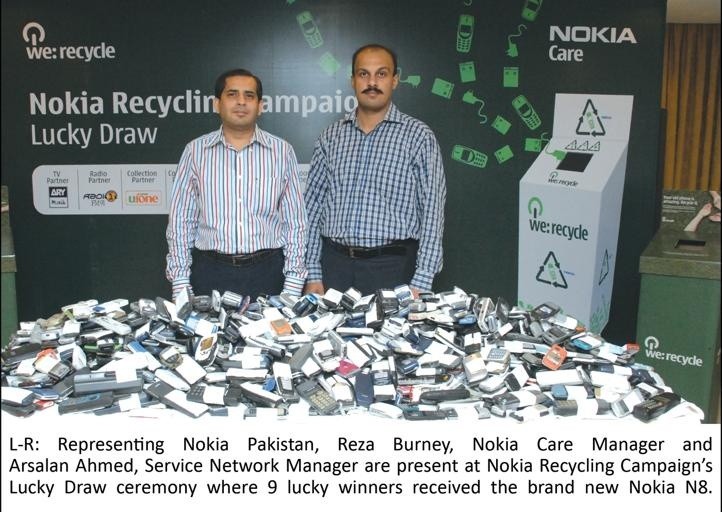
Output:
[191, 247, 280, 267]
[323, 239, 418, 259]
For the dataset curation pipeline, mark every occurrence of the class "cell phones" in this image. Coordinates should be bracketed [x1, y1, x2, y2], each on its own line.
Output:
[296, 11, 323, 48]
[456, 14, 474, 54]
[452, 144, 488, 168]
[522, 0, 544, 21]
[0, 285, 681, 421]
[512, 95, 541, 130]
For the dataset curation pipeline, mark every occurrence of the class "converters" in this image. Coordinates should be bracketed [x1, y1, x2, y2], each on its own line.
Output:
[509, 44, 518, 57]
[407, 76, 420, 86]
[462, 92, 476, 104]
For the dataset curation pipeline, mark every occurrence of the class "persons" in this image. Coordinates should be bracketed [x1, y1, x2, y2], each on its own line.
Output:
[166, 68, 310, 303]
[683, 191, 721, 231]
[303, 45, 447, 299]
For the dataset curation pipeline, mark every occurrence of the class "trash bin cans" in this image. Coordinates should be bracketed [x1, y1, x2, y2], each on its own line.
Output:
[636, 225, 721, 424]
